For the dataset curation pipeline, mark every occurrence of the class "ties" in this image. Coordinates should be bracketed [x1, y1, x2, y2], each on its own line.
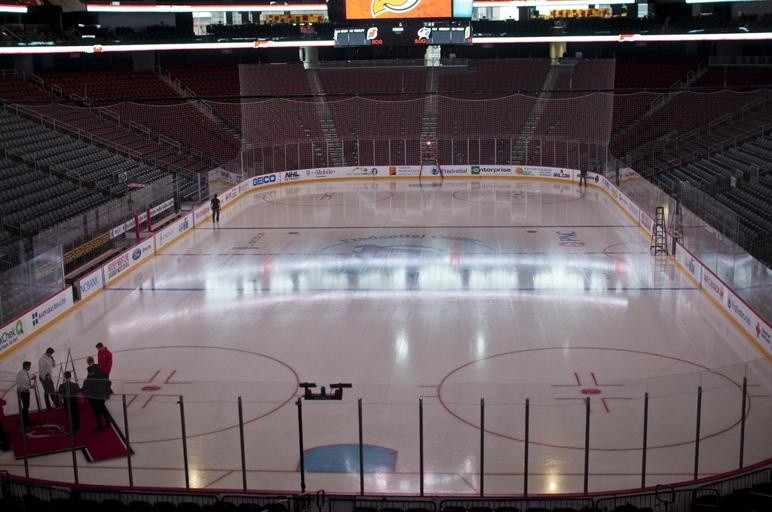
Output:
[26, 372, 32, 389]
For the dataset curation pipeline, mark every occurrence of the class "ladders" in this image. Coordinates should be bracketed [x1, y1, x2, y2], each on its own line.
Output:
[668, 206, 684, 247]
[650, 207, 668, 256]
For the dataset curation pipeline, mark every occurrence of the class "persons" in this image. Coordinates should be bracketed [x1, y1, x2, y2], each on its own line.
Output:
[95, 342, 114, 395]
[0, 399, 13, 451]
[15, 360, 36, 427]
[58, 371, 82, 431]
[38, 347, 60, 410]
[210, 194, 221, 222]
[82, 356, 114, 432]
[578, 163, 588, 187]
